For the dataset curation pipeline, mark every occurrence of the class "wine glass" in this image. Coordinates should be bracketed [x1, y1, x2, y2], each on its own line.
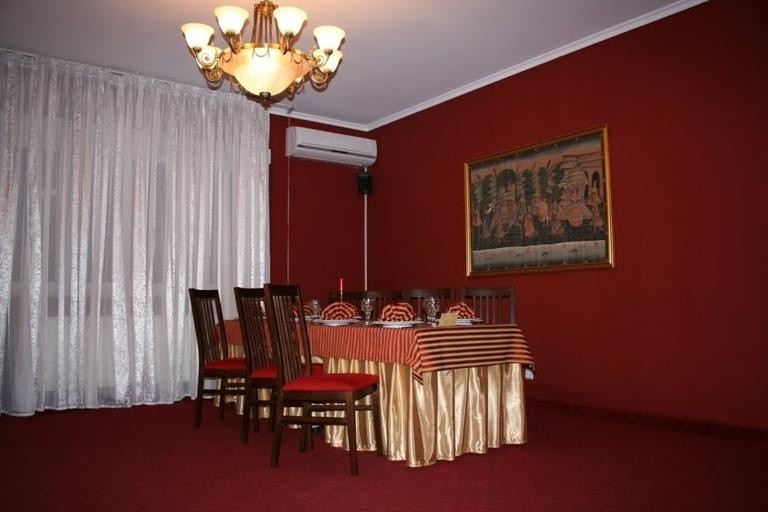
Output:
[360, 298, 376, 326]
[422, 295, 440, 328]
[308, 298, 322, 319]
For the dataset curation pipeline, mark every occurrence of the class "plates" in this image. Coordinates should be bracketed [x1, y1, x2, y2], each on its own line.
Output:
[312, 319, 360, 328]
[293, 315, 317, 323]
[371, 320, 424, 329]
[453, 318, 483, 325]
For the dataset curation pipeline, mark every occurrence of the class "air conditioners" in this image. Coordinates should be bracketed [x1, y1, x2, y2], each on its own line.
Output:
[285, 126, 378, 168]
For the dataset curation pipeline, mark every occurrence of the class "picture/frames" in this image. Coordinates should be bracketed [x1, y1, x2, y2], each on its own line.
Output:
[464, 123, 615, 279]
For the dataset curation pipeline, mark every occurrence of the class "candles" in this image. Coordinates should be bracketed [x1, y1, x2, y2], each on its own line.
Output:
[339, 278, 343, 292]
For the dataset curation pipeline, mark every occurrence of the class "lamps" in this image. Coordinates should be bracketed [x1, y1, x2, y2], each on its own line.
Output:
[180, 0, 346, 110]
